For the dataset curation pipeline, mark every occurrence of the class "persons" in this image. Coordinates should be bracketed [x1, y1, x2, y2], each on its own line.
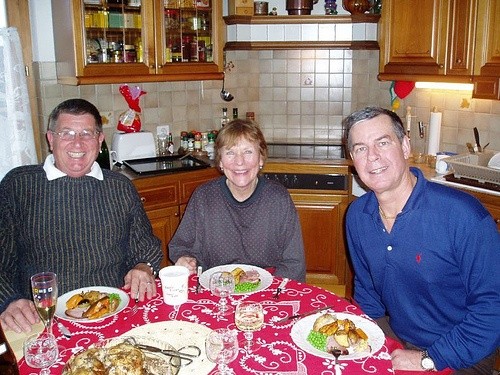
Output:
[0, 99, 163, 335]
[343, 107, 500, 375]
[168, 119, 307, 283]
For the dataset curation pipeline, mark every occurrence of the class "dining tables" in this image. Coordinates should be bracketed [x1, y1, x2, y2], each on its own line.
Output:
[18, 274, 455, 375]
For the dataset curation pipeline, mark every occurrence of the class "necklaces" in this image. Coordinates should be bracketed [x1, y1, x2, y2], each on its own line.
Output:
[379, 205, 397, 219]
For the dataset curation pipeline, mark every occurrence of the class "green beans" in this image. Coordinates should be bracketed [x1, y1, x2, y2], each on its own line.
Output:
[109, 293, 121, 303]
[307, 330, 327, 350]
[235, 282, 258, 293]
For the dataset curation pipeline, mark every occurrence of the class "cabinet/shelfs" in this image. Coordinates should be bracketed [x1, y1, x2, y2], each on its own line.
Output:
[378, 0, 500, 101]
[51, 0, 225, 86]
[133, 167, 222, 264]
[263, 162, 353, 300]
[223, 13, 379, 50]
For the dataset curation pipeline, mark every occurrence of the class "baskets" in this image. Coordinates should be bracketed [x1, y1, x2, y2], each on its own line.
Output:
[442, 151, 500, 186]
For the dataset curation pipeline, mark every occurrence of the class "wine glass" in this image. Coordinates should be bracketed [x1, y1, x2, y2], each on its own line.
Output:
[31, 272, 59, 333]
[209, 271, 236, 316]
[204, 328, 240, 375]
[235, 301, 264, 352]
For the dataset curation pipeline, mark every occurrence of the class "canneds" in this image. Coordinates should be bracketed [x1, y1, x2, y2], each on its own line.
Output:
[88, 41, 137, 64]
[165, 12, 210, 63]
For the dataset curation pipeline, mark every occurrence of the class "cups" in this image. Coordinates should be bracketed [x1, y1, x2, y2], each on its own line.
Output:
[159, 265, 190, 306]
[24, 334, 59, 368]
[428, 151, 460, 174]
[254, 0, 318, 16]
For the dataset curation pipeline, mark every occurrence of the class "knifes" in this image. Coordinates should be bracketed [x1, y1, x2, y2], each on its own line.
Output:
[196, 266, 202, 294]
[53, 319, 71, 338]
[278, 304, 335, 324]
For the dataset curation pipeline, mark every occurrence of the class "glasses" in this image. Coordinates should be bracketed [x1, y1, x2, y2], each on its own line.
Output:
[52, 128, 101, 140]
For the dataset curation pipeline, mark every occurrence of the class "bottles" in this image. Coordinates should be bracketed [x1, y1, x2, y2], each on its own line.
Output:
[169, 106, 256, 162]
[323, 0, 382, 15]
[83, 0, 214, 64]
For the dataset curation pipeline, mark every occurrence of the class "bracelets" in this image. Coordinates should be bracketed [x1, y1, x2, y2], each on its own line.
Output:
[147, 262, 158, 277]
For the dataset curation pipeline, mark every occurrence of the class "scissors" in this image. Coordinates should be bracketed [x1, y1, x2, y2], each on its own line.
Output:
[138, 344, 201, 368]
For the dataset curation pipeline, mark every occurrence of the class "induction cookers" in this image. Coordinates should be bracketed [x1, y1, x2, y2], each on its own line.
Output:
[255, 143, 347, 191]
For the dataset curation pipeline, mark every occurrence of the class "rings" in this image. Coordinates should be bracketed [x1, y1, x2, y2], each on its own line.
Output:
[147, 282, 152, 285]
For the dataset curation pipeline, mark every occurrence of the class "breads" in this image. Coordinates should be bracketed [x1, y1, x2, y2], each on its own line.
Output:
[67, 343, 149, 375]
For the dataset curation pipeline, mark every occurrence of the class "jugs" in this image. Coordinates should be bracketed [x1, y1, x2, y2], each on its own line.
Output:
[411, 120, 429, 163]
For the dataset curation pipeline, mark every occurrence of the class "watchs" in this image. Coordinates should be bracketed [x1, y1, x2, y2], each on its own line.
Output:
[421, 349, 435, 372]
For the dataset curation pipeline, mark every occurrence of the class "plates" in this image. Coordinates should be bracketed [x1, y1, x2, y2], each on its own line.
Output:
[62, 335, 181, 375]
[199, 264, 273, 296]
[291, 312, 386, 360]
[54, 286, 130, 323]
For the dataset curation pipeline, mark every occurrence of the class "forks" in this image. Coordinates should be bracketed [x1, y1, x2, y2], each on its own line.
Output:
[130, 292, 139, 316]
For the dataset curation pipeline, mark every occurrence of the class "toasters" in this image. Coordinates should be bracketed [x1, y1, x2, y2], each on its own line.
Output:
[108, 129, 158, 167]
